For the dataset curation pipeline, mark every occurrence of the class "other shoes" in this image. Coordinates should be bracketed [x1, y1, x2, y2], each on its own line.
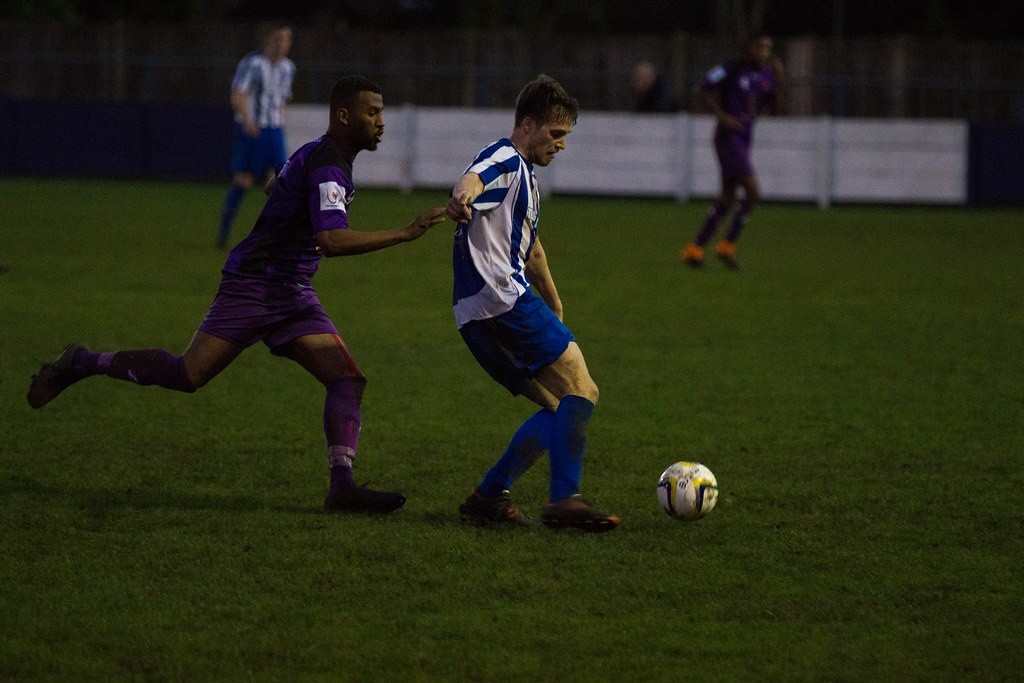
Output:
[684, 242, 712, 273]
[716, 239, 738, 268]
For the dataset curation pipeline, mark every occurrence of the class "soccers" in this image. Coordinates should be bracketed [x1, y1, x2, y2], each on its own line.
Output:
[656, 460, 719, 522]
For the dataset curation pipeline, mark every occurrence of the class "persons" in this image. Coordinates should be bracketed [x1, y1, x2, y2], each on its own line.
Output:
[681, 29, 784, 269]
[622, 62, 687, 113]
[26, 73, 446, 512]
[445, 74, 620, 534]
[215, 18, 298, 247]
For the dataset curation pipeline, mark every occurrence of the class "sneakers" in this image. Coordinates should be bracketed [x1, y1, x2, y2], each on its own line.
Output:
[27, 343, 88, 409]
[458, 490, 542, 528]
[325, 480, 406, 515]
[539, 494, 621, 532]
